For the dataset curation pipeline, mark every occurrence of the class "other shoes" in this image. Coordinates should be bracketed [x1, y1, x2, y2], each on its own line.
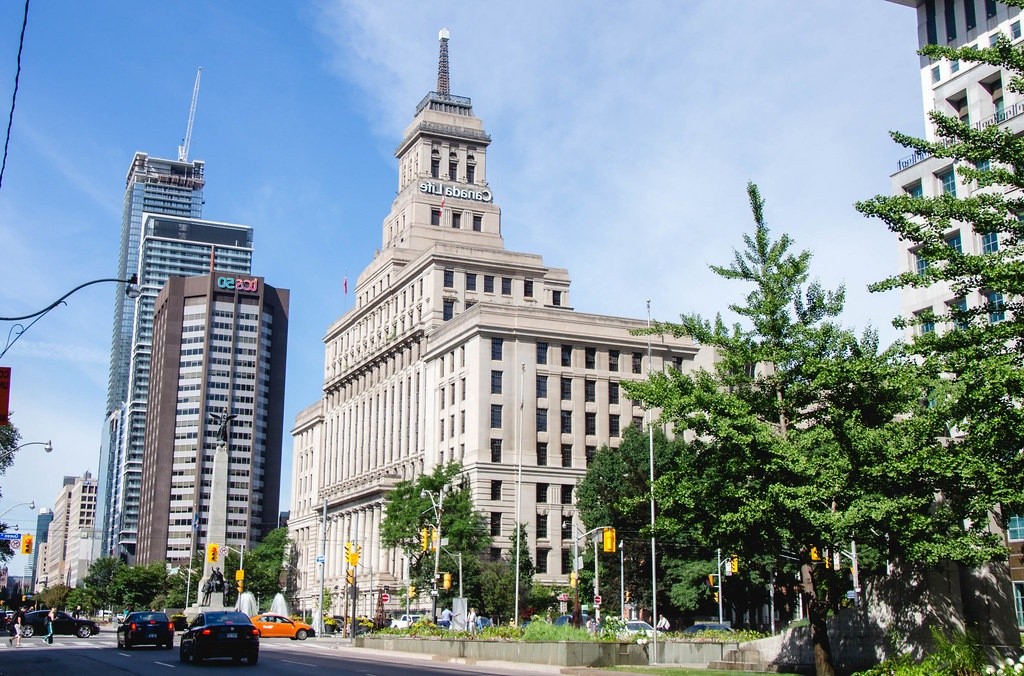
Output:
[9, 639, 12, 645]
[42, 638, 46, 644]
[48, 643, 56, 646]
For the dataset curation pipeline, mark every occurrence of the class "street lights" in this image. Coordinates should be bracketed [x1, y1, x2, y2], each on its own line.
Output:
[562, 521, 600, 623]
[420, 490, 443, 624]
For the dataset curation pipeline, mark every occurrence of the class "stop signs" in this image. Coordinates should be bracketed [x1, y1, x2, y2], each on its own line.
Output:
[382, 594, 389, 602]
[594, 596, 601, 604]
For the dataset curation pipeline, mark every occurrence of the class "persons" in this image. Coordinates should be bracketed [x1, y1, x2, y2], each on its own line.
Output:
[586, 616, 601, 630]
[123, 606, 135, 618]
[547, 614, 552, 624]
[202, 566, 231, 607]
[656, 614, 671, 631]
[468, 608, 482, 634]
[27, 606, 34, 612]
[441, 604, 460, 629]
[9, 605, 27, 647]
[41, 608, 58, 645]
[489, 616, 494, 627]
[151, 607, 156, 612]
[75, 606, 81, 619]
[509, 618, 515, 625]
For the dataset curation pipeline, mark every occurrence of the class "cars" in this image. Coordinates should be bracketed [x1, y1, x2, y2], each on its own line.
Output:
[250, 612, 316, 640]
[117, 611, 174, 649]
[598, 621, 662, 637]
[179, 610, 259, 665]
[0, 610, 100, 639]
[554, 614, 600, 631]
[682, 624, 732, 633]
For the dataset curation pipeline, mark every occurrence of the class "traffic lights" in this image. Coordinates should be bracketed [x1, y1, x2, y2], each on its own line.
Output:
[237, 582, 243, 593]
[706, 575, 713, 586]
[343, 543, 353, 584]
[21, 535, 32, 554]
[714, 592, 718, 602]
[419, 528, 427, 551]
[409, 586, 415, 597]
[207, 544, 218, 562]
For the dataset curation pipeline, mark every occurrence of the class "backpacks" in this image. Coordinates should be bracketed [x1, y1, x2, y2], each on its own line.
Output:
[11, 611, 20, 624]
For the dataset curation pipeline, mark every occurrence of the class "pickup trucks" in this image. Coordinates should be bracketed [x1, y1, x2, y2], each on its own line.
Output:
[390, 615, 421, 629]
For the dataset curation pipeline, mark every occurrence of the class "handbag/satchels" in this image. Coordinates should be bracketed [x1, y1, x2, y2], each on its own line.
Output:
[43, 616, 48, 626]
